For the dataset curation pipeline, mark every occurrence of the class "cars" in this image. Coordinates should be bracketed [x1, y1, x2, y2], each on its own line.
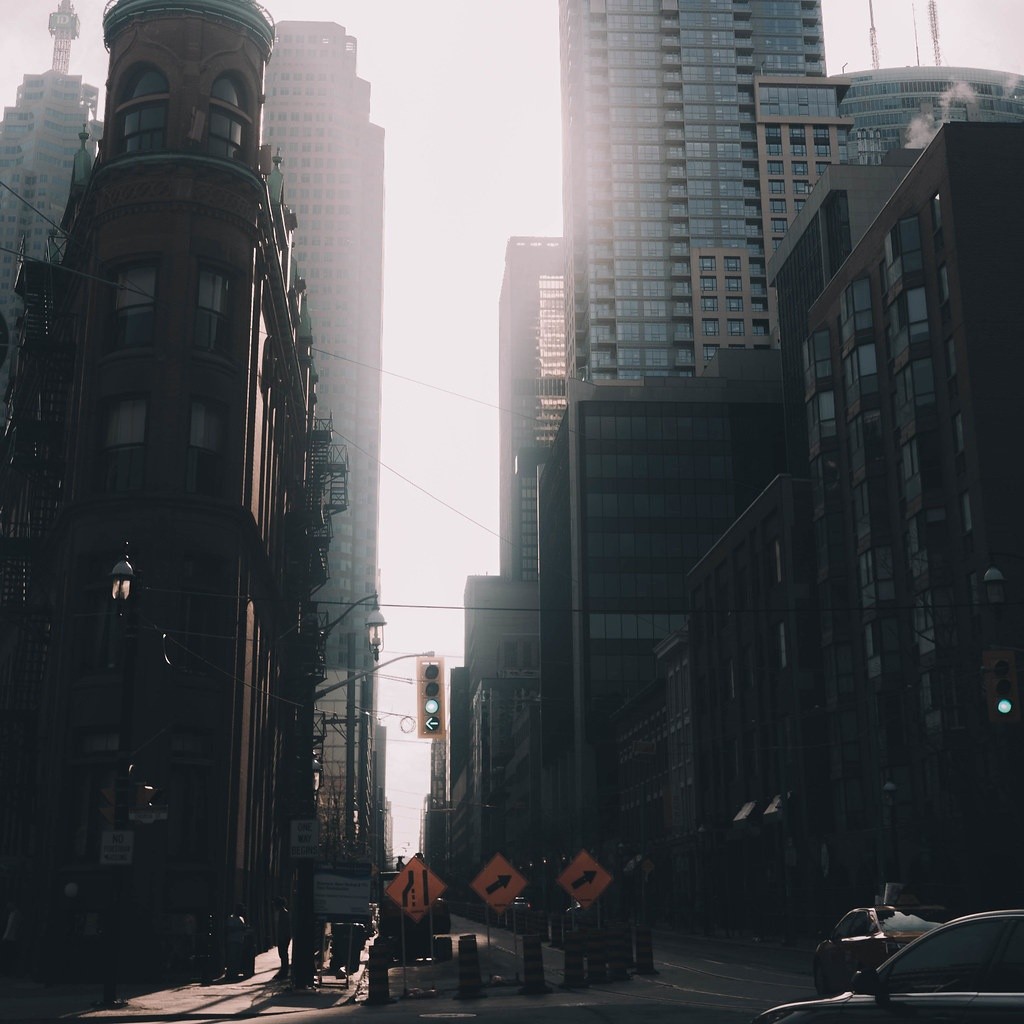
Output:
[750, 909, 1024, 1024]
[813, 904, 994, 1003]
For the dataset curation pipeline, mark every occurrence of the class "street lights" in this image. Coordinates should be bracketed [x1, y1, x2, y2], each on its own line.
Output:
[292, 588, 385, 987]
[98, 544, 139, 1008]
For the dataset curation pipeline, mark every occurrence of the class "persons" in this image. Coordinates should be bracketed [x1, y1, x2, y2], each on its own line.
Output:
[0, 902, 25, 979]
[566, 894, 581, 930]
[225, 902, 250, 979]
[272, 894, 291, 981]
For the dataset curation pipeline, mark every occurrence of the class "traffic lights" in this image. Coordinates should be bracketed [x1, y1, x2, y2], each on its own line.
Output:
[418, 659, 447, 739]
